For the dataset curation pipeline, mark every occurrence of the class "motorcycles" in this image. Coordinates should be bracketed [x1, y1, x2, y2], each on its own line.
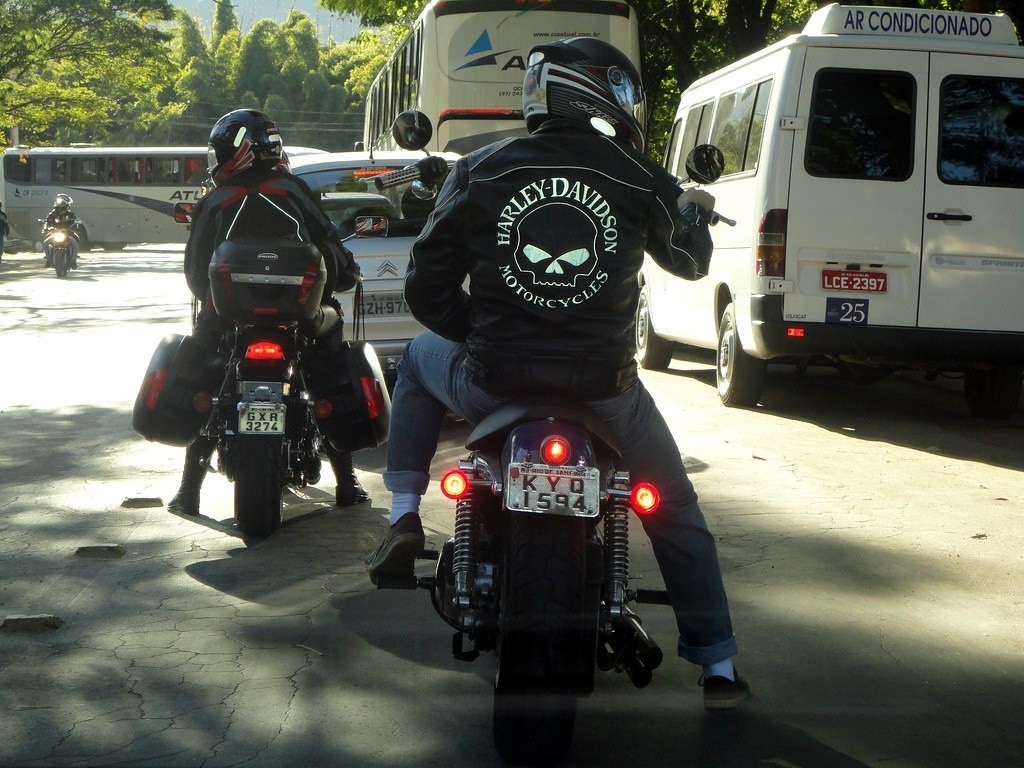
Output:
[358, 108, 737, 757]
[37, 218, 81, 277]
[131, 200, 391, 530]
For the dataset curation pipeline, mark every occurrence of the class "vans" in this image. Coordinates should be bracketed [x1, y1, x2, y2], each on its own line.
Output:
[635, 2, 1024, 408]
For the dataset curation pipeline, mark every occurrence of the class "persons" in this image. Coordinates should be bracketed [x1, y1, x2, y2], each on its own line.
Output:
[167, 108, 368, 515]
[366, 37, 750, 709]
[0, 202, 10, 263]
[43, 194, 80, 270]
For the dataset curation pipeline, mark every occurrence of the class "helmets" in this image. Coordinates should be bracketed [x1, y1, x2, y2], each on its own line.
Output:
[208, 108, 288, 187]
[54, 199, 69, 216]
[523, 35, 648, 154]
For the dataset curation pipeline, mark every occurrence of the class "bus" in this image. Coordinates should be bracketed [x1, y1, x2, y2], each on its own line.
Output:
[0, 143, 329, 254]
[363, 0, 640, 155]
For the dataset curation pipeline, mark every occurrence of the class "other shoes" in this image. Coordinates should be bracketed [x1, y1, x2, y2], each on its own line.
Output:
[369, 512, 425, 583]
[45, 259, 50, 268]
[335, 480, 370, 506]
[71, 259, 78, 269]
[698, 665, 749, 709]
[169, 491, 200, 517]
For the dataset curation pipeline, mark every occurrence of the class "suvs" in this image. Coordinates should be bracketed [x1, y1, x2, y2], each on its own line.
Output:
[287, 152, 465, 375]
